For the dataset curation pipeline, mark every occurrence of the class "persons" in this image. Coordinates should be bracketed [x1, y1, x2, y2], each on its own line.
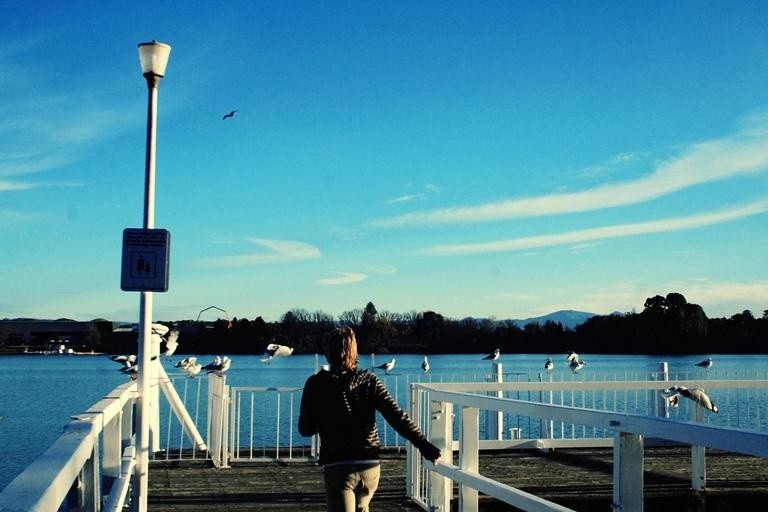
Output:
[297, 326, 443, 512]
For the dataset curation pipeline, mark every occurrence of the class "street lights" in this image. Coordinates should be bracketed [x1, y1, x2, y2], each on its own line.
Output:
[136, 40, 171, 512]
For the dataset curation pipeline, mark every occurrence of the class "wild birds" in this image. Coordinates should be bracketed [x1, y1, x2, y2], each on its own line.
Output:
[174, 353, 233, 377]
[482, 348, 502, 361]
[108, 352, 139, 377]
[219, 110, 240, 122]
[694, 357, 714, 373]
[374, 358, 396, 375]
[421, 355, 430, 374]
[259, 343, 293, 365]
[668, 385, 719, 413]
[544, 350, 585, 371]
